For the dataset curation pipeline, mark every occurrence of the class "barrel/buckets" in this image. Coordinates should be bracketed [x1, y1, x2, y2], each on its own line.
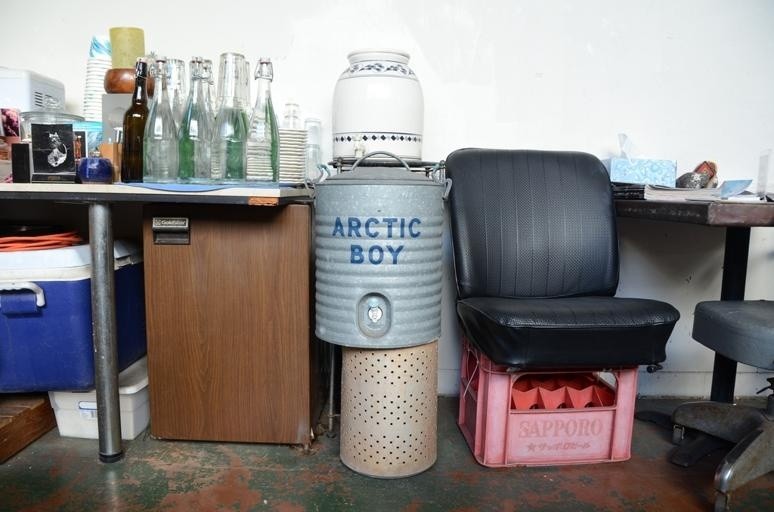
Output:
[311, 165, 451, 349]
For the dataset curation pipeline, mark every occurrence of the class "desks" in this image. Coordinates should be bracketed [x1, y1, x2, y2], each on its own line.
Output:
[0, 180, 314, 463]
[615, 197, 774, 470]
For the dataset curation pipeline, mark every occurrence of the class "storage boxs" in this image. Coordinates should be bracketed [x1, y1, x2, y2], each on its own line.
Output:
[0, 235, 149, 442]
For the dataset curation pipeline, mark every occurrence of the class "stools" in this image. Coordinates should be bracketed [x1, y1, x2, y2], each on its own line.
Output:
[670, 297, 774, 511]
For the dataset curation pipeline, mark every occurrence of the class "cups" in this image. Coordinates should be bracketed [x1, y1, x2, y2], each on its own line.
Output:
[83, 22, 114, 124]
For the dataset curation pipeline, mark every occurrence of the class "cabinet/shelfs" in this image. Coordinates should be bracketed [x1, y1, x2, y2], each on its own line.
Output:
[140, 201, 328, 454]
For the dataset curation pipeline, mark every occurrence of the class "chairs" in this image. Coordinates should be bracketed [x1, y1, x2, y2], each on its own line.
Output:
[445, 147, 681, 372]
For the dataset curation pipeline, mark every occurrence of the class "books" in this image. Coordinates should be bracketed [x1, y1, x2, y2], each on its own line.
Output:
[611, 181, 722, 204]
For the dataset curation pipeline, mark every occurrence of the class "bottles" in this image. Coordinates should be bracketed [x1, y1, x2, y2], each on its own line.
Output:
[119, 54, 322, 188]
